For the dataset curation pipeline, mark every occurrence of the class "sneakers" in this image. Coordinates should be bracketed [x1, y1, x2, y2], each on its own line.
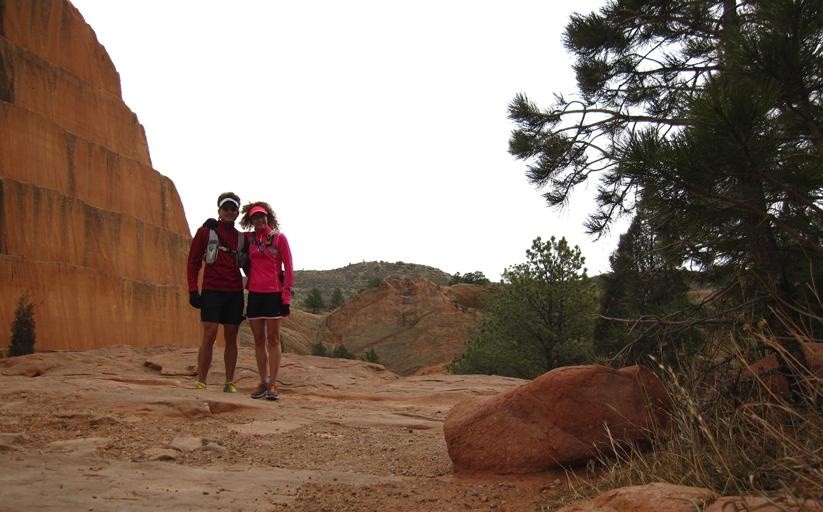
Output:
[249, 381, 280, 401]
[221, 381, 237, 393]
[195, 380, 209, 392]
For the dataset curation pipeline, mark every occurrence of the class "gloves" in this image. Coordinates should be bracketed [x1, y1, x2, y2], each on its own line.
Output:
[189, 290, 202, 308]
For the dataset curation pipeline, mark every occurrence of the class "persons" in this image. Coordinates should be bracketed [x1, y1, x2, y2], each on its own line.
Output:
[187, 191, 247, 393]
[203, 201, 293, 400]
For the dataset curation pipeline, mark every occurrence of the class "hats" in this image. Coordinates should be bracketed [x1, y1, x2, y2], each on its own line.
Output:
[218, 197, 239, 208]
[248, 206, 268, 217]
